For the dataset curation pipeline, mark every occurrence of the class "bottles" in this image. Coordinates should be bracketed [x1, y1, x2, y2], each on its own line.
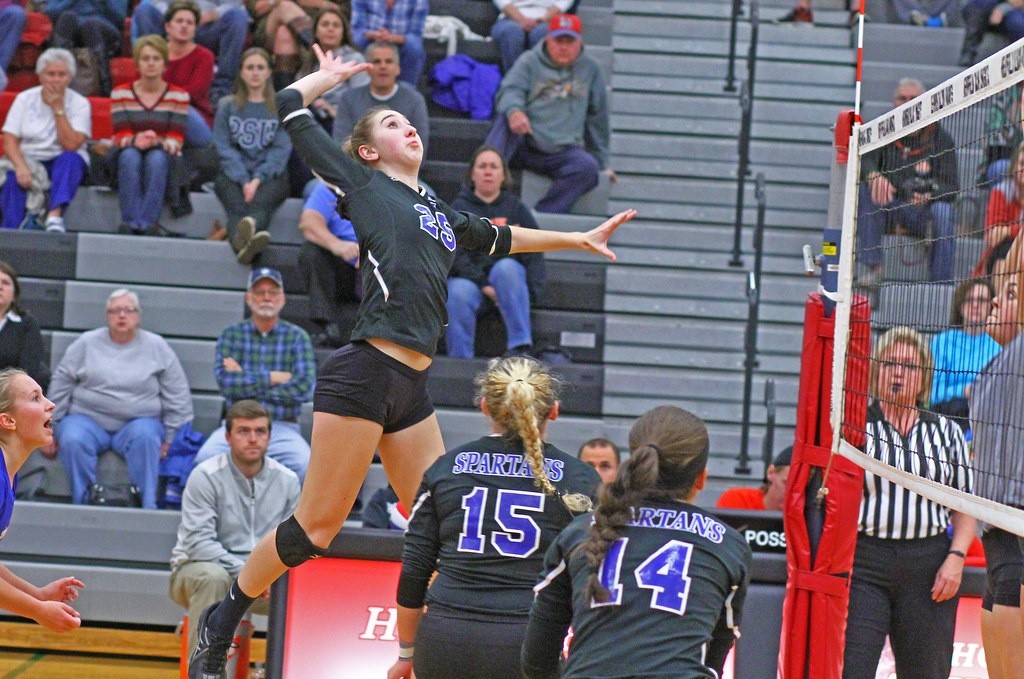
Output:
[254, 662, 266, 679]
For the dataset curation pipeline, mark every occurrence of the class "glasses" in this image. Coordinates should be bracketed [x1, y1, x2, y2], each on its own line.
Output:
[964, 297, 989, 303]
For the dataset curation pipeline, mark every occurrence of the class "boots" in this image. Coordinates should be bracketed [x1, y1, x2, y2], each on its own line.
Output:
[959, 6, 991, 66]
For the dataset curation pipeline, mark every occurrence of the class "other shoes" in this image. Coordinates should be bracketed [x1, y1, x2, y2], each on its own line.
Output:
[232, 217, 270, 265]
[855, 287, 877, 309]
[45, 216, 66, 233]
[144, 224, 160, 235]
[118, 223, 135, 235]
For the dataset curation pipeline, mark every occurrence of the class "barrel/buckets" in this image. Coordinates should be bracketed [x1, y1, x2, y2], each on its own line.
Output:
[174, 611, 255, 679]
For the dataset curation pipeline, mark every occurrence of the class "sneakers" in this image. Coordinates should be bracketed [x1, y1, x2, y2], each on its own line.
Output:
[188, 601, 239, 679]
[850, 8, 869, 25]
[776, 5, 812, 27]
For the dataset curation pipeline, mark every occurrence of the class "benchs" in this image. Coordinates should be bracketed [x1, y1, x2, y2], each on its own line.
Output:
[0, 0, 1024, 630]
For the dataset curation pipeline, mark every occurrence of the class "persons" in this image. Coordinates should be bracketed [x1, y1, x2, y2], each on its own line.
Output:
[0, 0, 1024, 679]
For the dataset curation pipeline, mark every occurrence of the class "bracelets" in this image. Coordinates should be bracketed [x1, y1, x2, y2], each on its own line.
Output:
[54, 110, 66, 115]
[398, 640, 415, 661]
[949, 550, 967, 559]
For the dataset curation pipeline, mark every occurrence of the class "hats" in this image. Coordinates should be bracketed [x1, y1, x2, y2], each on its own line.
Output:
[763, 445, 792, 484]
[249, 267, 282, 287]
[549, 15, 582, 41]
